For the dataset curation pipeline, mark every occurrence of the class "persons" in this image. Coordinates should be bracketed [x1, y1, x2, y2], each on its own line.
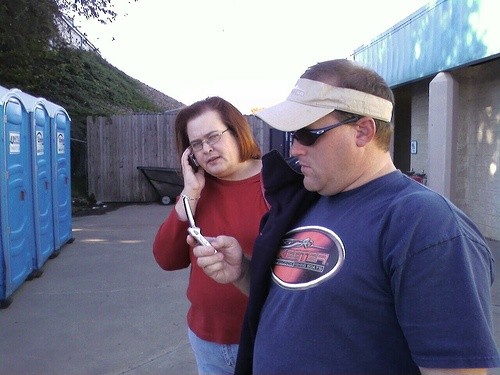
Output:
[151, 96, 271, 374]
[186, 58, 500, 375]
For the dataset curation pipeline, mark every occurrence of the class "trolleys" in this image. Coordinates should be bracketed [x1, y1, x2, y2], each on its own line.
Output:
[138, 167, 185, 205]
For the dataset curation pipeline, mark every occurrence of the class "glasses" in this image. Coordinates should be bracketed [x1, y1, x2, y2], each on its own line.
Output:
[189, 128, 230, 152]
[291, 115, 363, 147]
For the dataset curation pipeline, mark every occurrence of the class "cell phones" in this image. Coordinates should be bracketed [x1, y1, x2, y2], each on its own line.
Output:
[182, 194, 219, 254]
[188, 153, 199, 169]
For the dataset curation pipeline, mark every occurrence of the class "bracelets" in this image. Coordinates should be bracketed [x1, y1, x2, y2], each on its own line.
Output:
[179, 192, 201, 201]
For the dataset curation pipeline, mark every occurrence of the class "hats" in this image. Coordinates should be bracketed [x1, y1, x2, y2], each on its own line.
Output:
[252, 80, 394, 131]
